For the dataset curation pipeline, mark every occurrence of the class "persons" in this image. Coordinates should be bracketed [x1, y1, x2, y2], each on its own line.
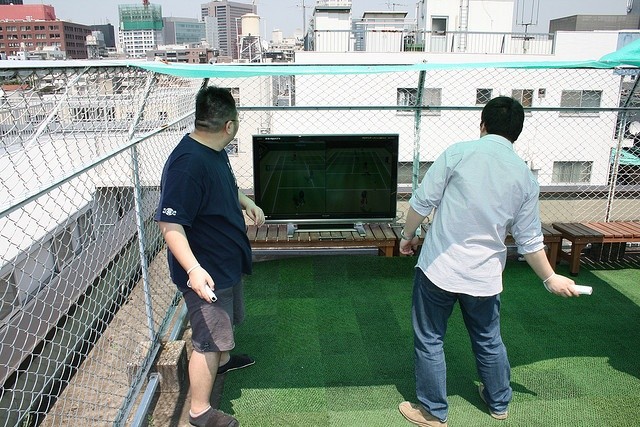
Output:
[396, 97, 580, 427]
[153, 86, 265, 426]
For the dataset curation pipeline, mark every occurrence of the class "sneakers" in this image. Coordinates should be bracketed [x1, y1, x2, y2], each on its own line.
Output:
[478, 382, 509, 419]
[398, 401, 448, 427]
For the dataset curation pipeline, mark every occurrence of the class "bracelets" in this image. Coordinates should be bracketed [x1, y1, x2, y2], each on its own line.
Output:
[400, 228, 416, 240]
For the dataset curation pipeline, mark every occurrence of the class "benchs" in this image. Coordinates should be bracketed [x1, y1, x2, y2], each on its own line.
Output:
[552, 220, 640, 278]
[392, 221, 562, 271]
[244, 224, 397, 258]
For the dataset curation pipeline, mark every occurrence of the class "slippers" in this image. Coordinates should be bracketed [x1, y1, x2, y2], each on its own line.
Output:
[217, 354, 255, 376]
[188, 407, 239, 426]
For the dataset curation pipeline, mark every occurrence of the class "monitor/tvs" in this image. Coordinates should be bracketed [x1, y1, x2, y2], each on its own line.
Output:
[253, 133, 400, 233]
